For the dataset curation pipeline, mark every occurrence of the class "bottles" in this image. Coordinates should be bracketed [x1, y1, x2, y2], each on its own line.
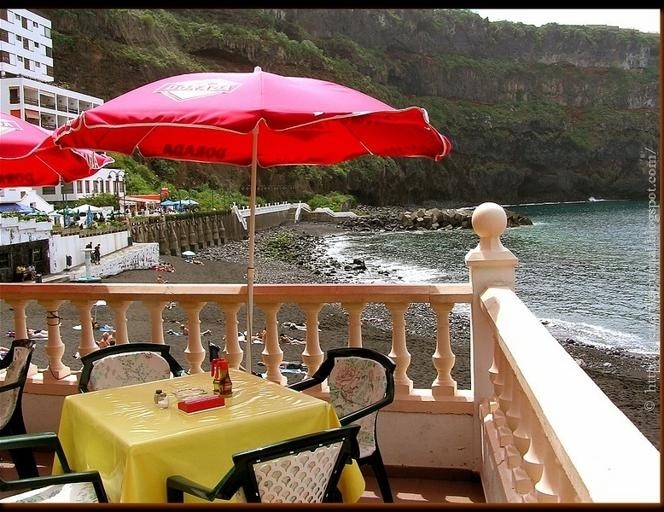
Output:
[153, 389, 162, 405]
[157, 392, 169, 410]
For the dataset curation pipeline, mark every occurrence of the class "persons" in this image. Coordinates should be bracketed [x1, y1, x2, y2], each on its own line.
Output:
[164, 301, 212, 337]
[184, 258, 204, 266]
[15, 263, 37, 281]
[237, 321, 308, 374]
[0, 318, 115, 373]
[148, 262, 175, 272]
[85, 241, 101, 265]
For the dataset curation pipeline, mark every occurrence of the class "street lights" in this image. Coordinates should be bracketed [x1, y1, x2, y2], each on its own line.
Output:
[105, 169, 127, 220]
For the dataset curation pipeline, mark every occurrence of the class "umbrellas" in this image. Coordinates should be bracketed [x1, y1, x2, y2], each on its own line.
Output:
[161, 199, 199, 206]
[92, 300, 107, 322]
[51, 67, 452, 374]
[181, 251, 195, 256]
[0, 110, 115, 190]
[70, 204, 103, 225]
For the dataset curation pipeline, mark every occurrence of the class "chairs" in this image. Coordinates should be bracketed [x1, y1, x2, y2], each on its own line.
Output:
[78, 344, 182, 393]
[0, 340, 38, 489]
[0, 432, 109, 503]
[289, 347, 396, 503]
[166, 423, 360, 503]
